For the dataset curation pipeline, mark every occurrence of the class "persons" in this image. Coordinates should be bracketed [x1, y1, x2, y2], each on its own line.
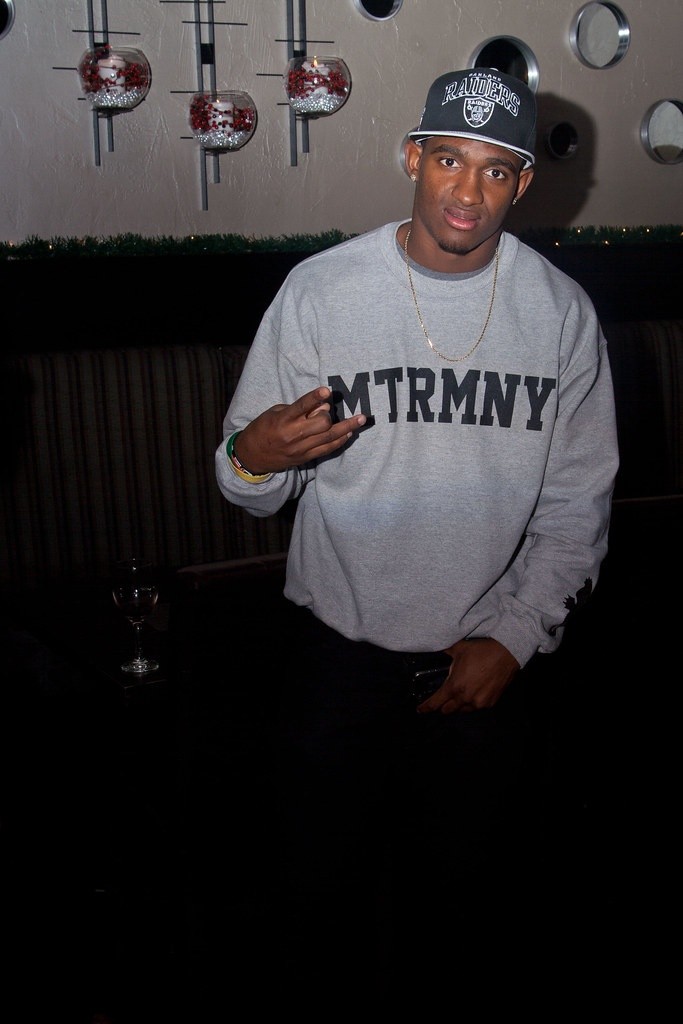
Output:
[212, 68, 621, 717]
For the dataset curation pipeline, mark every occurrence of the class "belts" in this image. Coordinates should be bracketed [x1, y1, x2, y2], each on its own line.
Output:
[287, 615, 451, 701]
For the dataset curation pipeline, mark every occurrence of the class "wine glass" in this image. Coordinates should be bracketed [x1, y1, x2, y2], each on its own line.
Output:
[110, 558, 162, 672]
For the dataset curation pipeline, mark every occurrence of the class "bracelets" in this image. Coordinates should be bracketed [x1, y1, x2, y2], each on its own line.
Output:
[226, 432, 271, 483]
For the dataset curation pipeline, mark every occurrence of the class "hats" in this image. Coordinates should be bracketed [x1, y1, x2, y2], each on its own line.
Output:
[407, 67, 539, 169]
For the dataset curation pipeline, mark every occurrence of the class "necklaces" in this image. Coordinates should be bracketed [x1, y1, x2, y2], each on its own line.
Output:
[404, 229, 499, 362]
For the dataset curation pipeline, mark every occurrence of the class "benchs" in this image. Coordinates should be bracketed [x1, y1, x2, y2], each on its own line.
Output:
[0, 319, 683, 587]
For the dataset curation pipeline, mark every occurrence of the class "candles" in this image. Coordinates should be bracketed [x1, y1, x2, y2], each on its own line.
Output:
[98, 55, 126, 91]
[206, 99, 234, 132]
[302, 60, 330, 94]
